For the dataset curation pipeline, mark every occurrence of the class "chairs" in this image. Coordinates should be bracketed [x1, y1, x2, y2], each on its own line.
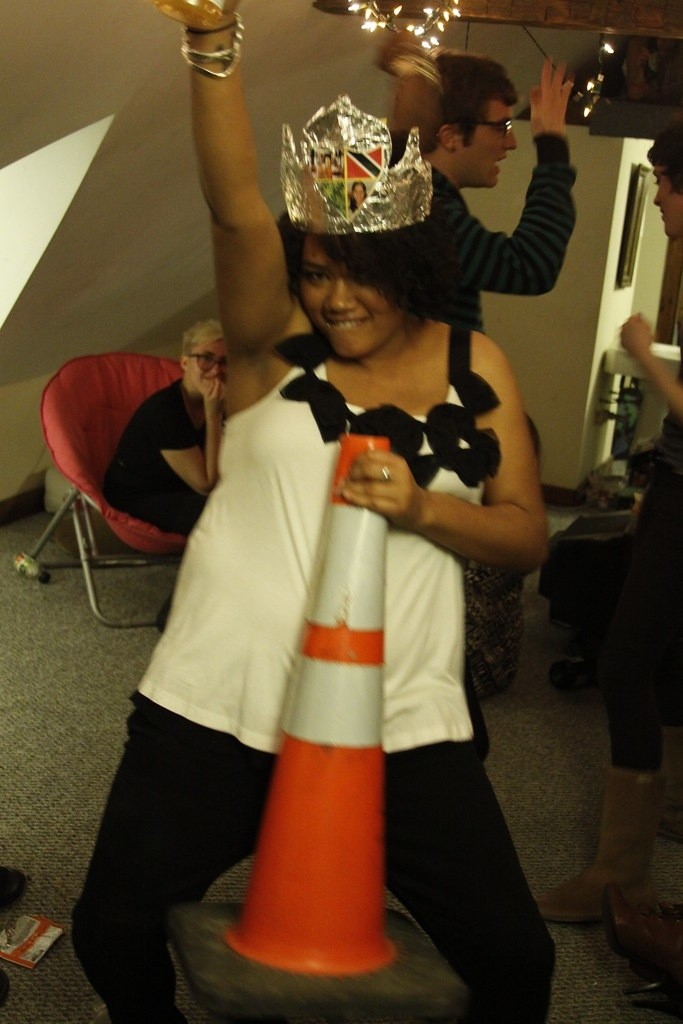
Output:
[31, 351, 187, 628]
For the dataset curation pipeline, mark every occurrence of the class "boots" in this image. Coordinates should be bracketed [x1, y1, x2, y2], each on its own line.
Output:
[657, 726, 683, 843]
[539, 762, 663, 922]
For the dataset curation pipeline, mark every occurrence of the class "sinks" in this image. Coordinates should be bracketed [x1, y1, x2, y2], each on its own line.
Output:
[605, 342, 682, 381]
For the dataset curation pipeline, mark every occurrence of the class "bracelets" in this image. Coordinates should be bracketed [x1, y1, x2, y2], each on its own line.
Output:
[180, 13, 243, 82]
[387, 53, 443, 88]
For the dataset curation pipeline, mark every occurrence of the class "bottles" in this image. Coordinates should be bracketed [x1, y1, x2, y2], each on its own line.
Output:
[10, 551, 53, 584]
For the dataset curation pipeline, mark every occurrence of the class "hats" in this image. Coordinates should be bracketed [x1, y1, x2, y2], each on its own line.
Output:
[279, 94, 433, 235]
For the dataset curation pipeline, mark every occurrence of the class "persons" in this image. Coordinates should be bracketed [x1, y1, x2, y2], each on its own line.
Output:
[316, 153, 370, 213]
[103, 319, 231, 633]
[602, 882, 682, 1017]
[530, 125, 682, 926]
[372, 30, 443, 157]
[69, 0, 557, 1024]
[420, 53, 576, 343]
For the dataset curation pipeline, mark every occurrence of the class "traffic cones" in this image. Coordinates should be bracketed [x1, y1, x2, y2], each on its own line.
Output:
[225, 434, 400, 976]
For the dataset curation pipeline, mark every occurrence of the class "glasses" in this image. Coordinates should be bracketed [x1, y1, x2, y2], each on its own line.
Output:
[478, 117, 514, 137]
[187, 353, 227, 371]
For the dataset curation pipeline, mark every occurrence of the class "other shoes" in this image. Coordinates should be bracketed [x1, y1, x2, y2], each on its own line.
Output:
[0, 866, 25, 912]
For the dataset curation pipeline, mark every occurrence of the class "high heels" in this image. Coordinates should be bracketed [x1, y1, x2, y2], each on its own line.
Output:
[609, 886, 683, 1023]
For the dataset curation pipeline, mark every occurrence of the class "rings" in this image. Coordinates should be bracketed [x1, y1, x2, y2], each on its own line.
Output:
[381, 466, 390, 479]
